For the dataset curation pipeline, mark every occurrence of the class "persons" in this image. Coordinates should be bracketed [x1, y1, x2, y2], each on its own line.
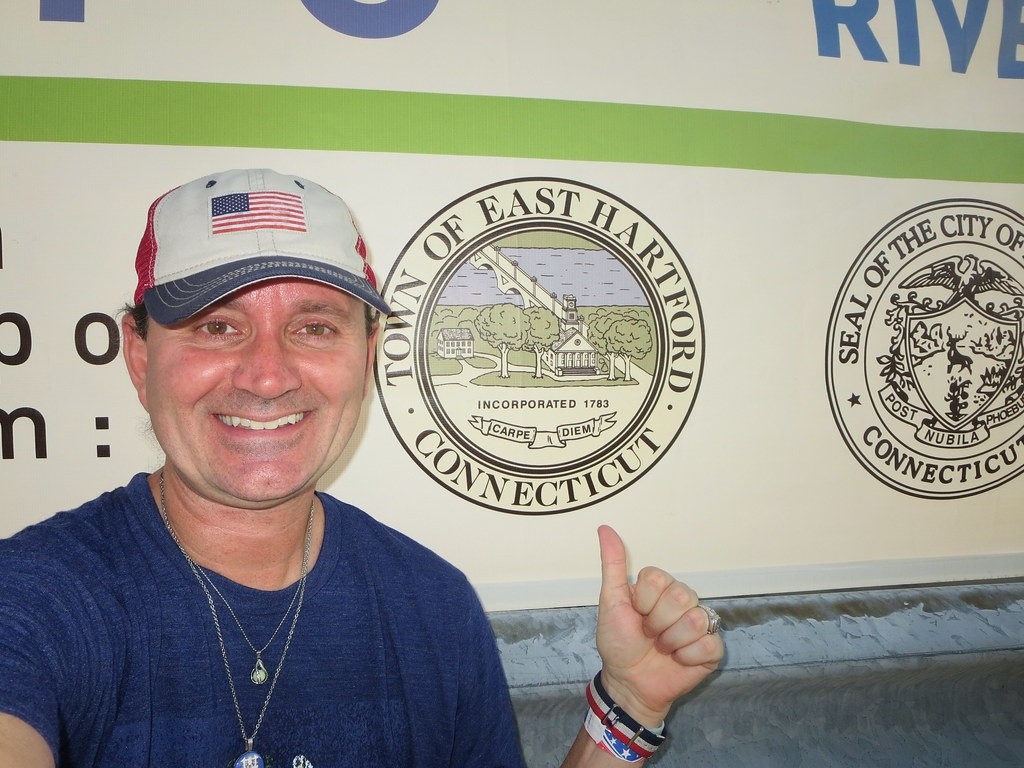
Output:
[0, 168, 725, 768]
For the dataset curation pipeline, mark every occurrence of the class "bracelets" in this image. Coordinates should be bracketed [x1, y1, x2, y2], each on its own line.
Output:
[583, 670, 666, 763]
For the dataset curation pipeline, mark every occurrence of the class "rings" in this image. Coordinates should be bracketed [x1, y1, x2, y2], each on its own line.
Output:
[697, 604, 722, 635]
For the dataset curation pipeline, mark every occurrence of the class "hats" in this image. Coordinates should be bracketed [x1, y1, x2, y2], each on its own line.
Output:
[135, 169, 391, 329]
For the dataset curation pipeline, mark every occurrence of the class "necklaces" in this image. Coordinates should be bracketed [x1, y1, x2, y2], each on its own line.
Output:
[160, 468, 314, 768]
[192, 556, 303, 685]
